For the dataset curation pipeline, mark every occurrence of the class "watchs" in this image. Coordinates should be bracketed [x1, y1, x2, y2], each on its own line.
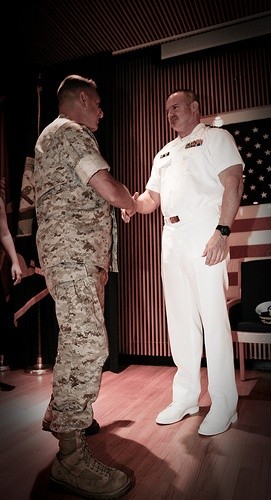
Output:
[216, 224, 231, 237]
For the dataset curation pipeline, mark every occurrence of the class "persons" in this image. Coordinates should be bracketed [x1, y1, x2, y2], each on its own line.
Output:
[33, 74, 137, 500]
[120, 89, 245, 436]
[0, 197, 23, 392]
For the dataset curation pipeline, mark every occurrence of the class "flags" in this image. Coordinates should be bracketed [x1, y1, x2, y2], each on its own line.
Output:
[0, 52, 116, 305]
[203, 116, 271, 334]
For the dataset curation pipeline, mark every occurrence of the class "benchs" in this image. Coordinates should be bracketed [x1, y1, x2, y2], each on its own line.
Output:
[226, 257, 271, 381]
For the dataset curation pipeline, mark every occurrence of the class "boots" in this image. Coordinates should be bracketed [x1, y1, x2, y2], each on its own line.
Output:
[42, 390, 101, 435]
[47, 432, 132, 500]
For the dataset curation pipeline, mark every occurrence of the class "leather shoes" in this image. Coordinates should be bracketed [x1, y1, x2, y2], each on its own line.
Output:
[155, 401, 199, 425]
[198, 404, 238, 436]
